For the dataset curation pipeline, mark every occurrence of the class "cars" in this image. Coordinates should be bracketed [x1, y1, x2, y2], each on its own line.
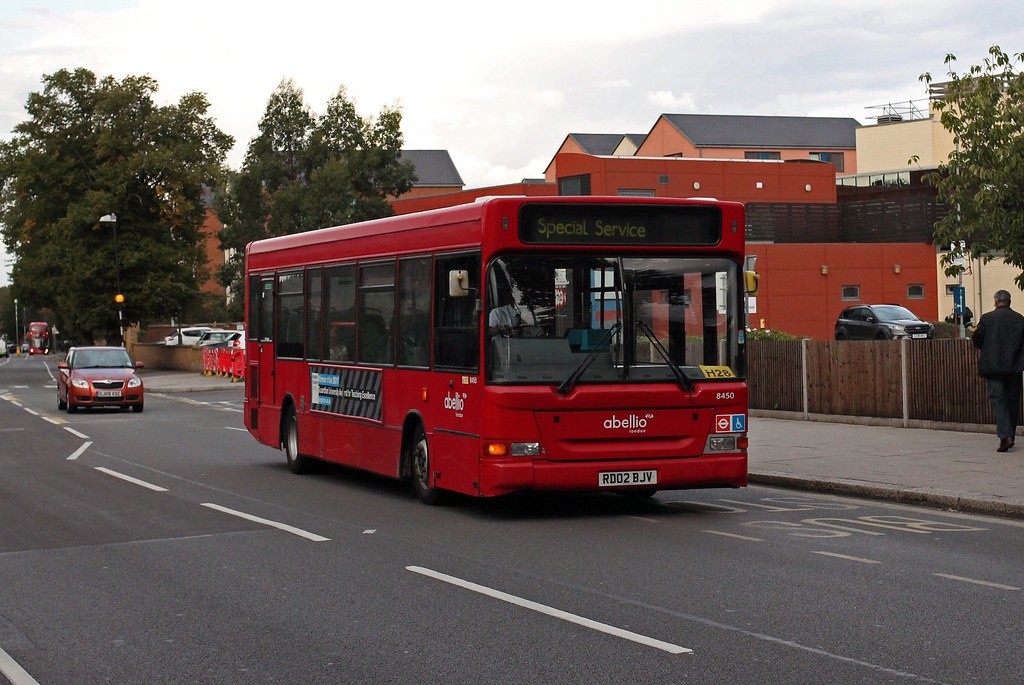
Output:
[57, 345, 145, 414]
[6, 340, 17, 353]
[164, 326, 245, 350]
[0, 339, 10, 358]
[20, 343, 30, 353]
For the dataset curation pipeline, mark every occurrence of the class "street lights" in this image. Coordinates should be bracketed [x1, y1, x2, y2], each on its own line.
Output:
[114, 294, 125, 347]
[99, 213, 121, 293]
[14, 298, 21, 356]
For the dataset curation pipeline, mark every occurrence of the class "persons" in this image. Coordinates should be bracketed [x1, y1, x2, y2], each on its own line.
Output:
[489, 274, 541, 341]
[972, 290, 1024, 451]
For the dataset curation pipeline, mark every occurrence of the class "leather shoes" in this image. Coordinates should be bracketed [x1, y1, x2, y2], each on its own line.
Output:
[997, 437, 1015, 452]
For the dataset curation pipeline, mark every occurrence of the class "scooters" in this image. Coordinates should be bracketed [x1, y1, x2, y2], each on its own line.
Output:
[945, 308, 973, 334]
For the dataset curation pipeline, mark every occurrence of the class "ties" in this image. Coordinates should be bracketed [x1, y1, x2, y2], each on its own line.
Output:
[517, 314, 522, 325]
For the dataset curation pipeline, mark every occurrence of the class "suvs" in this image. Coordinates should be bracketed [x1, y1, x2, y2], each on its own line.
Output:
[834, 303, 935, 340]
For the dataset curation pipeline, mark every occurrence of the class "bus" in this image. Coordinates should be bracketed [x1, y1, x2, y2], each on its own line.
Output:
[243, 195, 761, 508]
[27, 321, 51, 355]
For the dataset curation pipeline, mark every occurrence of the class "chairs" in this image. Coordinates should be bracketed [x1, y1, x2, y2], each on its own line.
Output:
[267, 304, 393, 364]
